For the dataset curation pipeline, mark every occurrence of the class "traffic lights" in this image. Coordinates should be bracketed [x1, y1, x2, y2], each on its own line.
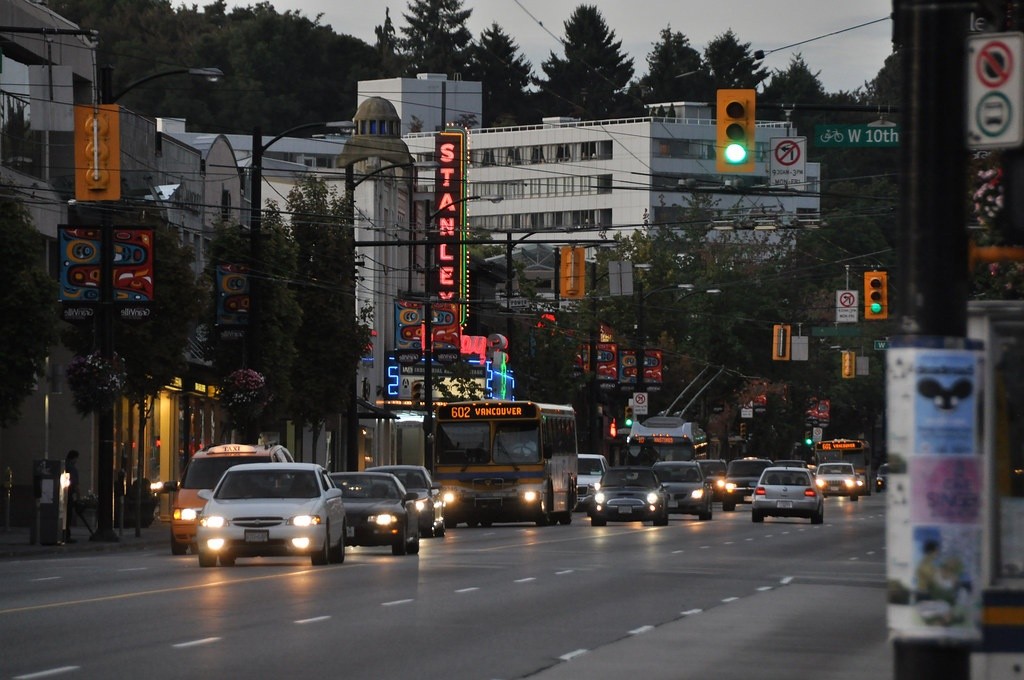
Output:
[863, 273, 888, 323]
[717, 88, 755, 175]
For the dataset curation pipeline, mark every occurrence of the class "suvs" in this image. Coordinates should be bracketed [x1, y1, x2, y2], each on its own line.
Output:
[366, 465, 447, 538]
[162, 439, 294, 554]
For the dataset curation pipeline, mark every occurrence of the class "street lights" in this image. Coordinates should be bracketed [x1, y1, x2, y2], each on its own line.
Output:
[422, 194, 503, 467]
[588, 261, 655, 452]
[90, 64, 224, 545]
[504, 231, 620, 373]
[635, 284, 694, 392]
[244, 117, 356, 441]
[338, 154, 439, 469]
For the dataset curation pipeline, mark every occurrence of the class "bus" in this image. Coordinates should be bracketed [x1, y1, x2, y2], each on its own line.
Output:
[433, 400, 580, 526]
[624, 418, 708, 464]
[815, 437, 875, 492]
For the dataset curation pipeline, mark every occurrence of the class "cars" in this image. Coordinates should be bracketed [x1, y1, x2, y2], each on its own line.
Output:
[329, 472, 421, 556]
[578, 453, 889, 513]
[195, 462, 347, 568]
[751, 466, 826, 526]
[588, 465, 670, 527]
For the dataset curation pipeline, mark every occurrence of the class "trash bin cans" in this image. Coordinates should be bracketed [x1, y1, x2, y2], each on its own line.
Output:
[31, 459, 70, 546]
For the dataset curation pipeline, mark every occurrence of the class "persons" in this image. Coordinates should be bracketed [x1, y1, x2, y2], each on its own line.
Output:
[512, 430, 537, 456]
[288, 473, 317, 499]
[684, 469, 699, 482]
[64, 451, 81, 544]
